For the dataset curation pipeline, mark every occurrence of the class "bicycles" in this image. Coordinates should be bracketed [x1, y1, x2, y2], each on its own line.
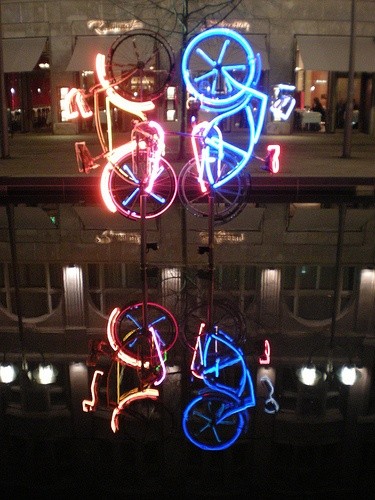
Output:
[81, 301, 179, 448]
[176, 28, 297, 225]
[63, 26, 178, 220]
[180, 300, 258, 452]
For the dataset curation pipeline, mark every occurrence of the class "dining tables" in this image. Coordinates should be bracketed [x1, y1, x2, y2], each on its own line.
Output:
[294, 108, 321, 132]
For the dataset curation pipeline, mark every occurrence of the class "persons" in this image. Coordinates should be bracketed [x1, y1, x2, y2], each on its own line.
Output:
[306, 93, 358, 130]
